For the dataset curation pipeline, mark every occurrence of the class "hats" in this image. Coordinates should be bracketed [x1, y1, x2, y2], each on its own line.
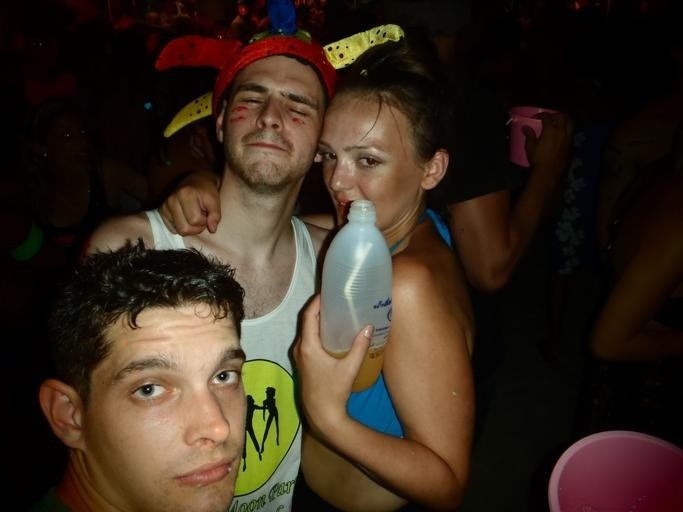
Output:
[153, 0, 404, 138]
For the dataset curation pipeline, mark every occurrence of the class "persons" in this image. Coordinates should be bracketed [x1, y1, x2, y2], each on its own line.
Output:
[2, 2, 681, 511]
